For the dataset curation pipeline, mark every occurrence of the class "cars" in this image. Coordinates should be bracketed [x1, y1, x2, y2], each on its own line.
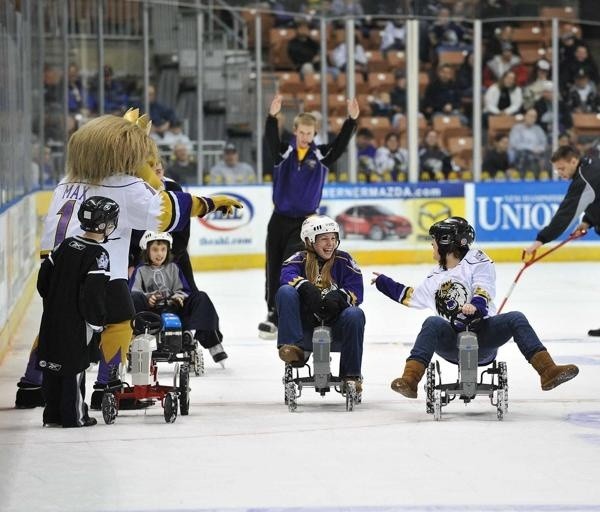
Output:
[334, 203, 412, 241]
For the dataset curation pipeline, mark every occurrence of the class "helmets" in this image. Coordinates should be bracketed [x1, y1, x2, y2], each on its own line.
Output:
[429, 216, 475, 254]
[139, 230, 173, 251]
[299, 213, 340, 247]
[77, 196, 119, 238]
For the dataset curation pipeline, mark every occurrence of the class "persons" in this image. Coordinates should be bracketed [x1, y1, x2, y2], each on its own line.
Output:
[125, 229, 229, 373]
[28, 60, 256, 182]
[35, 196, 123, 429]
[356, 126, 453, 182]
[521, 143, 599, 339]
[371, 215, 580, 400]
[126, 159, 199, 298]
[220, 0, 474, 113]
[256, 88, 359, 340]
[274, 214, 362, 396]
[482, 25, 598, 177]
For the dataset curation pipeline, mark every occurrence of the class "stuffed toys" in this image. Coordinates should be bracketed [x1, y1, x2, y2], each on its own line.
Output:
[15, 106, 244, 413]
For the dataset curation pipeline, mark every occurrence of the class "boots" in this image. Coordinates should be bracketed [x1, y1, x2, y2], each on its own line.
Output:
[390, 359, 425, 399]
[529, 350, 576, 392]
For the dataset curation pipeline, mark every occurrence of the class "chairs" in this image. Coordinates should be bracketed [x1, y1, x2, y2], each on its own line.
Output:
[235, 3, 599, 176]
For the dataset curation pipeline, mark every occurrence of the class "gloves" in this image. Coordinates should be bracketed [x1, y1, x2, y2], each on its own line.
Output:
[197, 190, 243, 219]
[323, 288, 352, 316]
[292, 279, 320, 311]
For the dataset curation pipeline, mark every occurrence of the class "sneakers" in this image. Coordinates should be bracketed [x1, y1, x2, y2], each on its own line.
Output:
[90, 379, 155, 410]
[41, 414, 97, 426]
[258, 313, 279, 340]
[183, 329, 193, 346]
[278, 344, 305, 368]
[15, 381, 49, 410]
[209, 343, 227, 362]
[341, 375, 362, 394]
